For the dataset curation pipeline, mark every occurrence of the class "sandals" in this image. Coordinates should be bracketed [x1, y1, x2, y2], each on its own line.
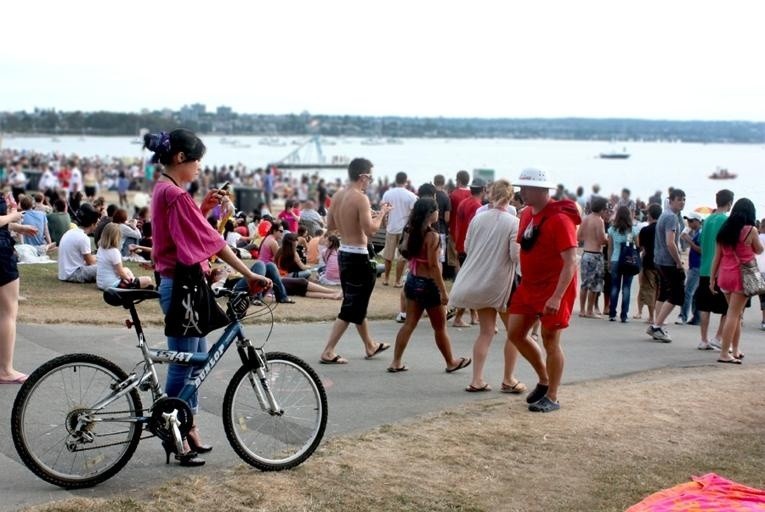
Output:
[466, 384, 491, 391]
[500, 382, 527, 393]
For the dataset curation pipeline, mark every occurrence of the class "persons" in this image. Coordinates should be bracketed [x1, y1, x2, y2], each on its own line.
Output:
[319, 158, 391, 366]
[141, 128, 275, 467]
[383, 171, 763, 330]
[709, 198, 763, 364]
[698, 189, 733, 353]
[0, 148, 416, 332]
[449, 180, 528, 394]
[645, 189, 685, 344]
[389, 197, 471, 373]
[0, 190, 39, 385]
[508, 168, 580, 413]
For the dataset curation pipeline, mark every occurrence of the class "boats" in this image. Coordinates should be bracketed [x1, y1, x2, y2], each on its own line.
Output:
[707, 169, 738, 180]
[223, 137, 404, 148]
[597, 153, 630, 160]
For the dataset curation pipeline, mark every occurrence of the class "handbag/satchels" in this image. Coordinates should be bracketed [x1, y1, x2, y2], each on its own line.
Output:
[740, 260, 765, 297]
[618, 242, 641, 275]
[165, 274, 231, 339]
[162, 433, 213, 466]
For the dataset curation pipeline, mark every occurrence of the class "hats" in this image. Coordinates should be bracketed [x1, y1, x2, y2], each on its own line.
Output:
[509, 167, 558, 189]
[468, 177, 488, 189]
[683, 211, 702, 222]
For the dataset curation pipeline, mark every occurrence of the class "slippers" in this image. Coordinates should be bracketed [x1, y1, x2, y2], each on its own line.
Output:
[716, 353, 744, 364]
[364, 343, 391, 360]
[579, 312, 668, 325]
[527, 383, 548, 403]
[387, 365, 409, 372]
[0, 374, 28, 384]
[529, 395, 560, 412]
[319, 355, 346, 364]
[446, 358, 471, 372]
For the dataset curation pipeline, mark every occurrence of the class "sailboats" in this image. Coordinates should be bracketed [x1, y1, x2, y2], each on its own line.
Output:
[270, 129, 373, 169]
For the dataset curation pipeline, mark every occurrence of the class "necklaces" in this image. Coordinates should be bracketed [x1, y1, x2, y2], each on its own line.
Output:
[162, 172, 182, 188]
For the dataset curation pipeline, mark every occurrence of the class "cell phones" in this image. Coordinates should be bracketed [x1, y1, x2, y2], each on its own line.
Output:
[217, 181, 231, 194]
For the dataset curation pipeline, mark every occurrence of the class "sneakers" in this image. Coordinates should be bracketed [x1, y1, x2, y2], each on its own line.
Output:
[382, 280, 403, 288]
[276, 297, 295, 303]
[252, 299, 262, 306]
[698, 336, 733, 353]
[396, 314, 406, 322]
[530, 332, 539, 341]
[675, 316, 701, 325]
[760, 322, 765, 330]
[645, 325, 672, 343]
[447, 308, 480, 327]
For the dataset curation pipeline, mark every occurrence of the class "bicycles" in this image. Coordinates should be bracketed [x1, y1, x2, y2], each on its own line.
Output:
[10, 277, 329, 490]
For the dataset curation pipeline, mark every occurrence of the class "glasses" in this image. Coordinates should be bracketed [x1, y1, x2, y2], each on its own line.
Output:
[358, 173, 373, 181]
[276, 229, 283, 233]
